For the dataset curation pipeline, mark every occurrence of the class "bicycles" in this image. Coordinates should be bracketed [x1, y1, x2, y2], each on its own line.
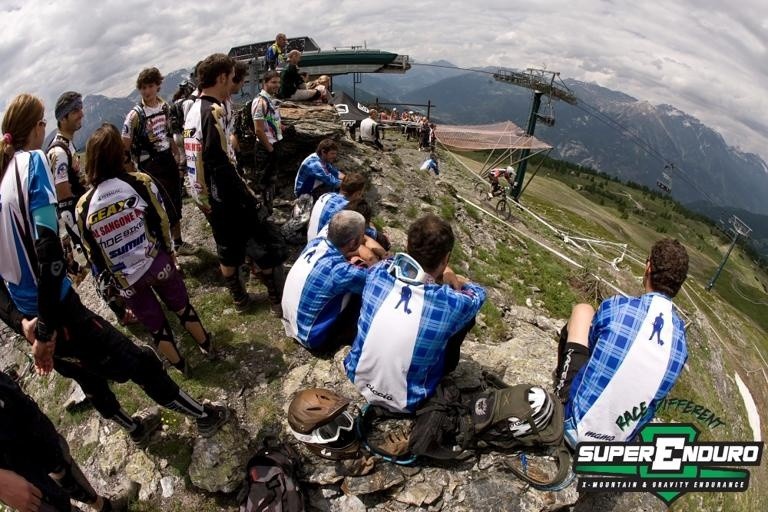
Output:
[474, 181, 517, 221]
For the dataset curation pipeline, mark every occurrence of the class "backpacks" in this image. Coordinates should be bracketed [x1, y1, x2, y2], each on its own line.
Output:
[408, 371, 570, 490]
[236, 436, 310, 511]
[281, 193, 314, 244]
[229, 94, 270, 152]
[168, 94, 197, 134]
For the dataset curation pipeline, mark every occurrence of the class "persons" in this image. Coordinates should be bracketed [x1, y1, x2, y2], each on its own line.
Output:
[172, 31, 440, 313]
[0, 93, 234, 449]
[488, 166, 516, 197]
[47, 90, 137, 328]
[343, 215, 488, 414]
[74, 118, 217, 375]
[281, 208, 368, 357]
[0, 369, 140, 512]
[121, 66, 199, 257]
[553, 237, 690, 456]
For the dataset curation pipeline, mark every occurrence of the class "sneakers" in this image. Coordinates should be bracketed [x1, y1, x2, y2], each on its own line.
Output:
[234, 292, 267, 311]
[129, 407, 161, 449]
[177, 359, 192, 379]
[199, 332, 216, 360]
[117, 309, 140, 326]
[196, 398, 230, 438]
[105, 481, 140, 510]
[488, 192, 494, 198]
[173, 241, 201, 256]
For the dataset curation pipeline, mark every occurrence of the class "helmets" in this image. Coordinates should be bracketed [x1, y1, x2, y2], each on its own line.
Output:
[507, 167, 515, 177]
[354, 403, 417, 465]
[246, 226, 290, 269]
[288, 388, 361, 460]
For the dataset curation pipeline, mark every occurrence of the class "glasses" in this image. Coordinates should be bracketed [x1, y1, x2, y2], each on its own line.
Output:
[387, 251, 435, 285]
[290, 410, 354, 444]
